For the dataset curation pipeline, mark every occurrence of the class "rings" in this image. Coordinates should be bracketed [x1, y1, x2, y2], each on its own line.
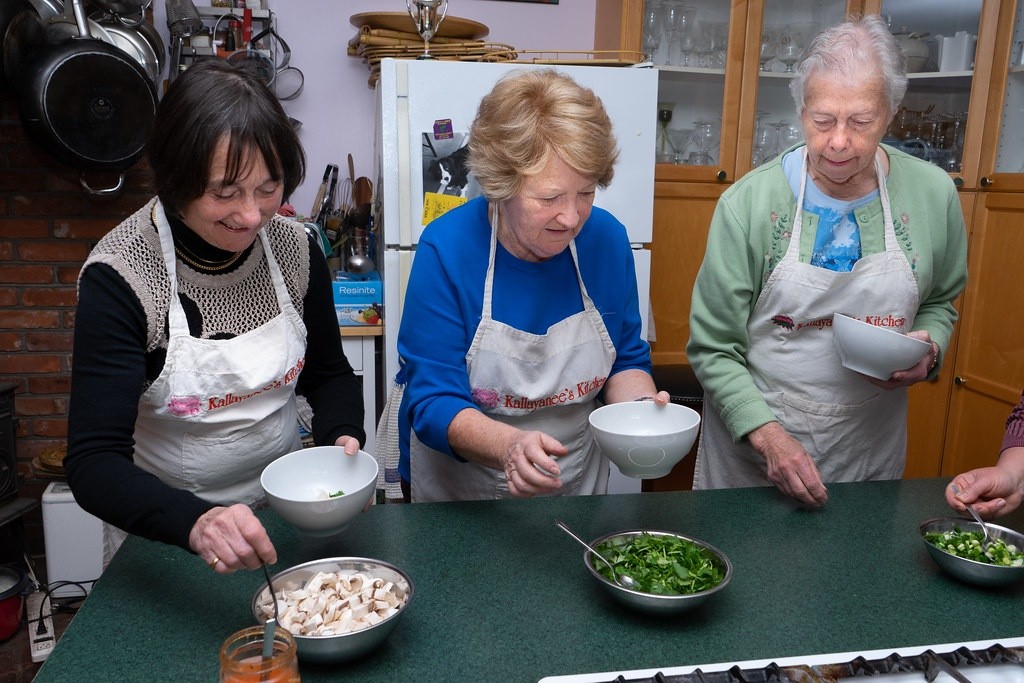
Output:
[510, 469, 518, 474]
[209, 557, 220, 570]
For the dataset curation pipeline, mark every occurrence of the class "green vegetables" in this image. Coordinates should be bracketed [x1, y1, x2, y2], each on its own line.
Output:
[328, 491, 344, 498]
[591, 530, 724, 596]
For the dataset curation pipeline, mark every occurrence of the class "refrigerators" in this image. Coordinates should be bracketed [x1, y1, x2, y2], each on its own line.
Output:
[374, 58, 659, 505]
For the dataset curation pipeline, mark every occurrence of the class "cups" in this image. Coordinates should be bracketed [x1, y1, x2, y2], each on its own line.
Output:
[342, 229, 376, 272]
[656, 154, 675, 162]
[779, 118, 801, 150]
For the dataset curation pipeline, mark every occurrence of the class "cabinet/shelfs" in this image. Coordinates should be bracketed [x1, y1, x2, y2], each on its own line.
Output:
[172, 0, 278, 72]
[595, 0, 1024, 491]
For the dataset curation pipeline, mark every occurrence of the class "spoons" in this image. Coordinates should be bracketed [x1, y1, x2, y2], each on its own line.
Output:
[556, 519, 642, 592]
[261, 559, 292, 636]
[952, 484, 996, 561]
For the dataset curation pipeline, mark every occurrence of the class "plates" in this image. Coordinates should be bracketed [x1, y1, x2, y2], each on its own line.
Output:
[350, 314, 359, 322]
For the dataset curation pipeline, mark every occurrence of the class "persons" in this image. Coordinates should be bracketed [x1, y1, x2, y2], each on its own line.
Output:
[946, 387, 1024, 519]
[684, 15, 970, 509]
[62, 54, 375, 576]
[396, 68, 671, 502]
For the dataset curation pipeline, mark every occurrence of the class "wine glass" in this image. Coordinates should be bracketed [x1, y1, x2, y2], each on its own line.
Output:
[642, 0, 821, 73]
[892, 107, 968, 172]
[664, 121, 720, 165]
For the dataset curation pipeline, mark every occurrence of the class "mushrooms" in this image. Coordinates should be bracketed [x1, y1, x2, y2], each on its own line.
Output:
[262, 570, 408, 637]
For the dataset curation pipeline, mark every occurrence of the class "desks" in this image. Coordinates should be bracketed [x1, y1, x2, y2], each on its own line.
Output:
[31, 478, 1024, 683]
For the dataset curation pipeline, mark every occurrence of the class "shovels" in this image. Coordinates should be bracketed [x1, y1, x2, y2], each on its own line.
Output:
[354, 176, 373, 207]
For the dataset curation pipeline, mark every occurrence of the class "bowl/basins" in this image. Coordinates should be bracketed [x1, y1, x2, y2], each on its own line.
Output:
[583, 528, 733, 608]
[252, 556, 415, 657]
[260, 446, 379, 537]
[832, 312, 932, 380]
[917, 517, 1024, 586]
[589, 402, 701, 478]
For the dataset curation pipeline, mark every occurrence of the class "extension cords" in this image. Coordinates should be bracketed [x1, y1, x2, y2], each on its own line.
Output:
[26, 591, 56, 662]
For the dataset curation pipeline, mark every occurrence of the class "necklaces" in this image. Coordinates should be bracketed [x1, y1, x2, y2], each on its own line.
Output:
[174, 245, 243, 273]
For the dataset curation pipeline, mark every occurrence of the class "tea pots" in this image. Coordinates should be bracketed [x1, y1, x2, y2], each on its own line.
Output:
[934, 30, 977, 71]
[881, 135, 929, 161]
[890, 25, 931, 73]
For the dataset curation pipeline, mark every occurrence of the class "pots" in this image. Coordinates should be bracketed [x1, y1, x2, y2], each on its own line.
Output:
[0, 0, 166, 194]
[0, 557, 36, 643]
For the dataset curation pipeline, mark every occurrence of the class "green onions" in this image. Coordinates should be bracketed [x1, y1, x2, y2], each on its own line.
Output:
[924, 523, 1024, 567]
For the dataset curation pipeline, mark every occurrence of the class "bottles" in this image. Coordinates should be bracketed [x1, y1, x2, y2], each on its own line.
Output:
[751, 112, 790, 167]
[221, 626, 300, 683]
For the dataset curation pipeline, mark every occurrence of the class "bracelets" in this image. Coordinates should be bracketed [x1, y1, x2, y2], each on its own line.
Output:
[931, 349, 939, 370]
